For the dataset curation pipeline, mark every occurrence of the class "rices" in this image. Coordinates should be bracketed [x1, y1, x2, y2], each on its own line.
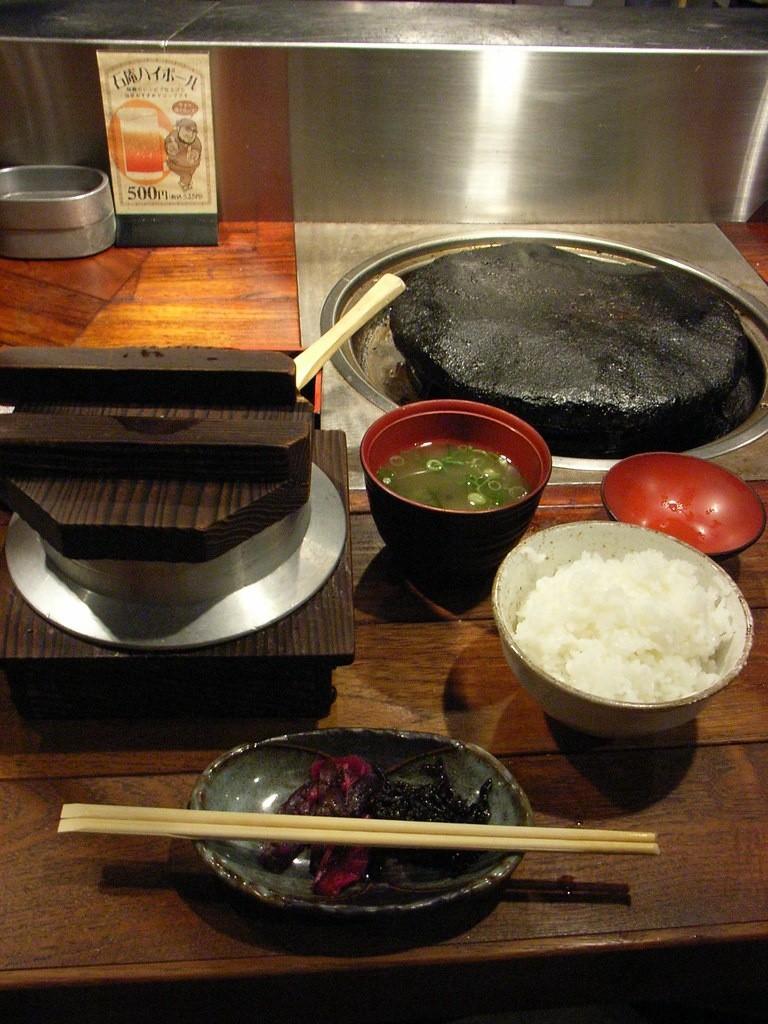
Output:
[515, 546, 736, 703]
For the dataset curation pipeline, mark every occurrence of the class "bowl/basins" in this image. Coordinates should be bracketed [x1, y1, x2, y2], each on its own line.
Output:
[360, 399, 553, 556]
[490, 520, 754, 737]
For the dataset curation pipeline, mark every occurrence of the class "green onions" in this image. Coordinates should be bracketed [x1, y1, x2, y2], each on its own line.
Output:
[374, 443, 530, 511]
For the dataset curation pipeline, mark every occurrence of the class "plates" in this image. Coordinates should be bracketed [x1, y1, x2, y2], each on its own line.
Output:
[189, 728, 535, 914]
[601, 453, 765, 563]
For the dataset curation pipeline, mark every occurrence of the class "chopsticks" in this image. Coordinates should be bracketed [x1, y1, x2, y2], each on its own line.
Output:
[58, 803, 662, 858]
